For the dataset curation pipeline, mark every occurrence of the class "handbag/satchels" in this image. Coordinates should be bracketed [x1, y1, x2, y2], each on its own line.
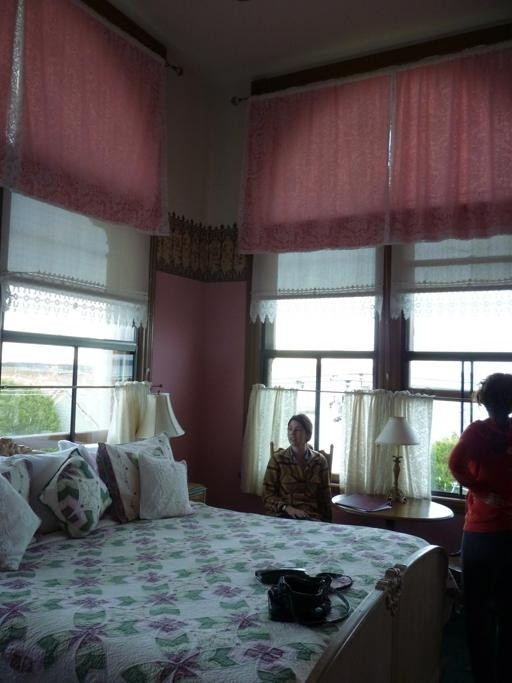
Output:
[256, 568, 311, 585]
[267, 575, 331, 625]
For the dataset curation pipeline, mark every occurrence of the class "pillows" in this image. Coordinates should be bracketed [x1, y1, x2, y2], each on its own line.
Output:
[0, 437, 195, 572]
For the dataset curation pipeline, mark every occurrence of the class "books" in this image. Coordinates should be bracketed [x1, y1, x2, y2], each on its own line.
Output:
[336, 492, 394, 511]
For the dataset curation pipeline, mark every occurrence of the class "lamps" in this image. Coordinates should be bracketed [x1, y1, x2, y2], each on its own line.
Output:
[135, 390, 187, 437]
[375, 415, 421, 505]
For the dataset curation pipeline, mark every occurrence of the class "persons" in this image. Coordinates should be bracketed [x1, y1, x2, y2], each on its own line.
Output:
[262, 414, 330, 522]
[448, 373, 512, 682]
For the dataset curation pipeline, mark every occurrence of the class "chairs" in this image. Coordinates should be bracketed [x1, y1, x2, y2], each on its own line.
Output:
[269, 441, 334, 488]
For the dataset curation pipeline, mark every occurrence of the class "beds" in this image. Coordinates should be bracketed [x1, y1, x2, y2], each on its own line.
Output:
[0, 436, 450, 682]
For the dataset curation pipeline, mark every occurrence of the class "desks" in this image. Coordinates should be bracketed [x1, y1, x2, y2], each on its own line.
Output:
[332, 493, 454, 547]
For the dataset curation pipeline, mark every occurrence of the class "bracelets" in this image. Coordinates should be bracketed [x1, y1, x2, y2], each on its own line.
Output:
[282, 504, 287, 511]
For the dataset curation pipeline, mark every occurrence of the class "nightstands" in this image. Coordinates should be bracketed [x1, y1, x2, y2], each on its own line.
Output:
[187, 481, 207, 504]
